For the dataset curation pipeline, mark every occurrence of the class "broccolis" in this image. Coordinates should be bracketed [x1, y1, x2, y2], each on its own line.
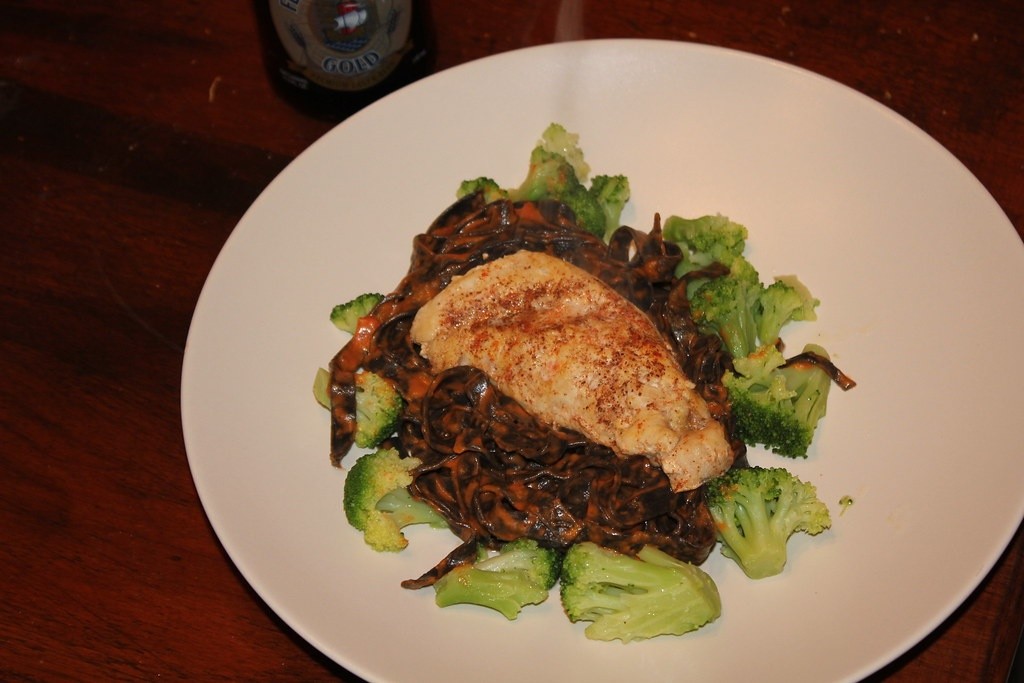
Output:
[317, 124, 832, 645]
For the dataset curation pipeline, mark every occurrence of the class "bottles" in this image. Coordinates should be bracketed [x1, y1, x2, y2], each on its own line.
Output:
[251, 1, 433, 117]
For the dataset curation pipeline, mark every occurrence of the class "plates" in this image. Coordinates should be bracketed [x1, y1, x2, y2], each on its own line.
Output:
[180, 38, 1024, 683]
